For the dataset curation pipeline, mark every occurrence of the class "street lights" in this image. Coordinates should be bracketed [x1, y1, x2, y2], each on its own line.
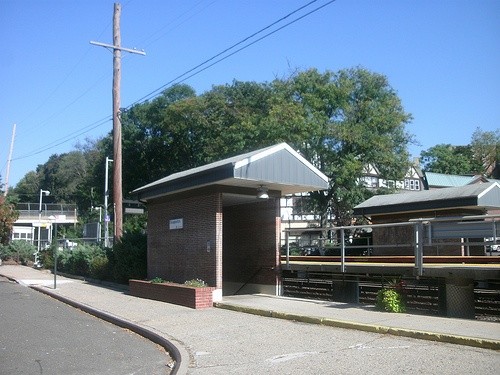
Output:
[39, 188, 50, 263]
